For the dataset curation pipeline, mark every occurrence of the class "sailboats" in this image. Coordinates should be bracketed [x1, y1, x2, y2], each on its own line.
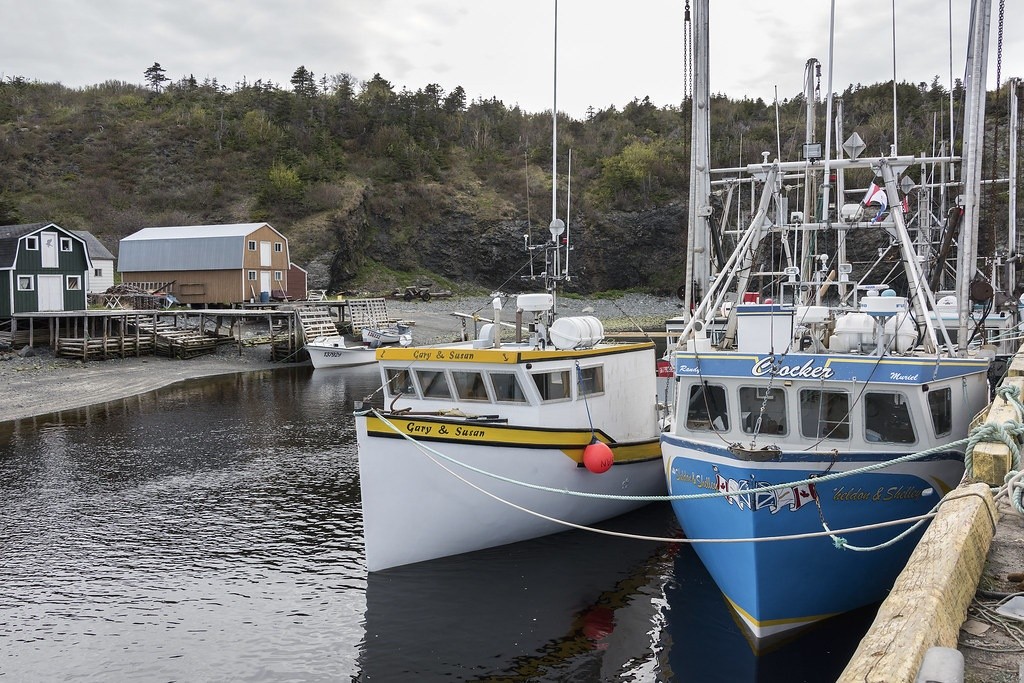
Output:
[672, 0, 1021, 663]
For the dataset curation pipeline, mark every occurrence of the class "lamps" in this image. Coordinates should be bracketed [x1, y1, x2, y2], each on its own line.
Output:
[838, 263, 853, 283]
[784, 267, 800, 283]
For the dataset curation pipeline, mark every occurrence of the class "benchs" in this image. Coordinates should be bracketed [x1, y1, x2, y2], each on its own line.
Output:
[272, 290, 293, 299]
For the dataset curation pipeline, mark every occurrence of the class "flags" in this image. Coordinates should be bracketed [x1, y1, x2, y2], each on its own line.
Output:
[899, 197, 908, 213]
[863, 183, 888, 223]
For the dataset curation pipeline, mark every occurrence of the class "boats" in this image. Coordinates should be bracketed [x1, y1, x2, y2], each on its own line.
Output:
[361, 313, 413, 345]
[351, 0, 669, 583]
[304, 336, 392, 369]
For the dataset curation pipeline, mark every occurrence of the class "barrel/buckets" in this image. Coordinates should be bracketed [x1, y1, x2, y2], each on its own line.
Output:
[261, 292, 269, 303]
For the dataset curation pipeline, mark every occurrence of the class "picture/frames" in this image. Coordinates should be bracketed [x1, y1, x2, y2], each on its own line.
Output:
[754, 388, 776, 402]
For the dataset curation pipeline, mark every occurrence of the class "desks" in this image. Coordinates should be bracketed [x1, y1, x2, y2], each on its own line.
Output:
[741, 412, 751, 431]
